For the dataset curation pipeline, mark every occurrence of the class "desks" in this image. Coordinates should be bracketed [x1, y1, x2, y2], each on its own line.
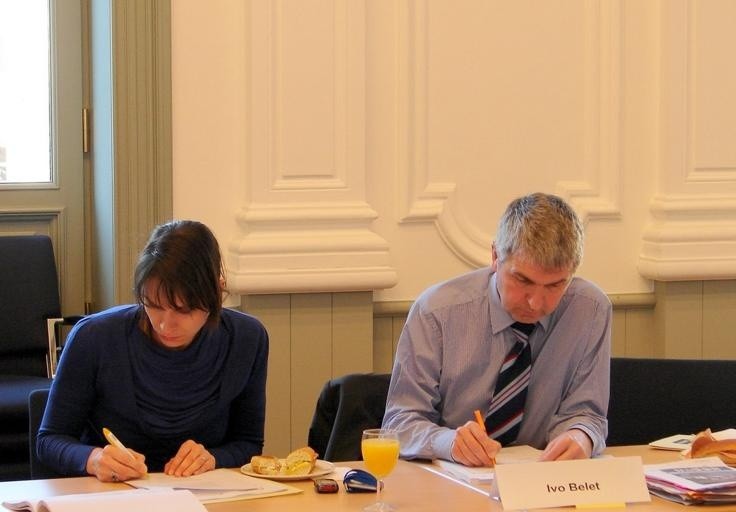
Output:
[0, 444, 733, 512]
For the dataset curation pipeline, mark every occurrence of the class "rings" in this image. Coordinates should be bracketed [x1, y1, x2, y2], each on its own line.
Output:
[111, 471, 119, 482]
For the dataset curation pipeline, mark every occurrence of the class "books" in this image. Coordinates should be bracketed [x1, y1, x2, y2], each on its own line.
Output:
[2, 486, 207, 512]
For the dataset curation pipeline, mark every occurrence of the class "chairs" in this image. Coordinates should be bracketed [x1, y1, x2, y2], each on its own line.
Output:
[0, 234, 75, 468]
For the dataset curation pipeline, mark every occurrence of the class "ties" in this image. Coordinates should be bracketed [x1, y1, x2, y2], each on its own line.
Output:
[486, 320, 541, 449]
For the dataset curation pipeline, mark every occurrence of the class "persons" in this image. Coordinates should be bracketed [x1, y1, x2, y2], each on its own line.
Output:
[378, 193, 612, 467]
[36, 221, 269, 482]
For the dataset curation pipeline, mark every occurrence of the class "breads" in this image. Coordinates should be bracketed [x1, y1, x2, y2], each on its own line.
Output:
[251, 455, 282, 475]
[285, 447, 319, 475]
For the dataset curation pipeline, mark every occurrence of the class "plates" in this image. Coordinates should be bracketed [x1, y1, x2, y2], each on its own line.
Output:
[239, 460, 335, 480]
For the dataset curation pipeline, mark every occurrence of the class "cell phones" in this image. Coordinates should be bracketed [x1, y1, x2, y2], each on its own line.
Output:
[314, 479, 339, 493]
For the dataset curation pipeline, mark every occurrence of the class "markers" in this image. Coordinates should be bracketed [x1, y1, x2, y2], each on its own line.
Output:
[474, 410, 494, 462]
[103, 427, 147, 480]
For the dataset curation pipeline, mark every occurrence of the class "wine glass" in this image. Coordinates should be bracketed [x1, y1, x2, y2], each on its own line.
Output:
[359, 430, 400, 512]
